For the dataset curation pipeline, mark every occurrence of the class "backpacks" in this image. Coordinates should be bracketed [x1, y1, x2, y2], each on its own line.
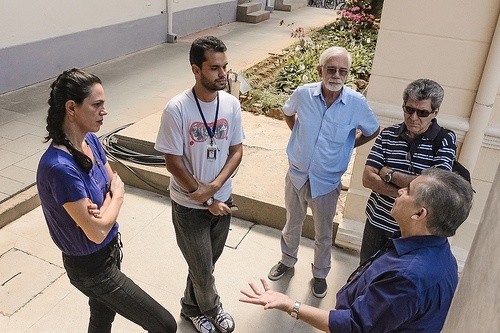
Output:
[433, 127, 472, 186]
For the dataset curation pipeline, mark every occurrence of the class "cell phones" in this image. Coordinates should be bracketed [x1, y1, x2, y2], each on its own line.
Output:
[227, 204, 239, 210]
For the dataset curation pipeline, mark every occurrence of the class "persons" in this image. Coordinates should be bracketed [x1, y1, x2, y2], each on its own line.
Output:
[359, 79, 458, 266]
[267, 48, 380, 297]
[155, 37, 246, 333]
[239, 168, 473, 333]
[36, 69, 177, 333]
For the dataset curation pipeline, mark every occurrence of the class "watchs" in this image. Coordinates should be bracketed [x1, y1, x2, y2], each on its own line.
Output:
[385, 169, 395, 182]
[203, 196, 215, 207]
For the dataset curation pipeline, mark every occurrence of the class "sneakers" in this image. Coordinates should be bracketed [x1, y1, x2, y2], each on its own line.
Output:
[268, 262, 294, 280]
[180, 309, 216, 333]
[204, 302, 236, 333]
[312, 276, 327, 298]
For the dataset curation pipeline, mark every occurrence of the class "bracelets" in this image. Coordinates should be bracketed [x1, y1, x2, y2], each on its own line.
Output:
[288, 301, 301, 320]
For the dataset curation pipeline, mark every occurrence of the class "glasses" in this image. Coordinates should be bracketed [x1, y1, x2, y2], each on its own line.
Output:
[401, 105, 433, 117]
[322, 65, 348, 76]
